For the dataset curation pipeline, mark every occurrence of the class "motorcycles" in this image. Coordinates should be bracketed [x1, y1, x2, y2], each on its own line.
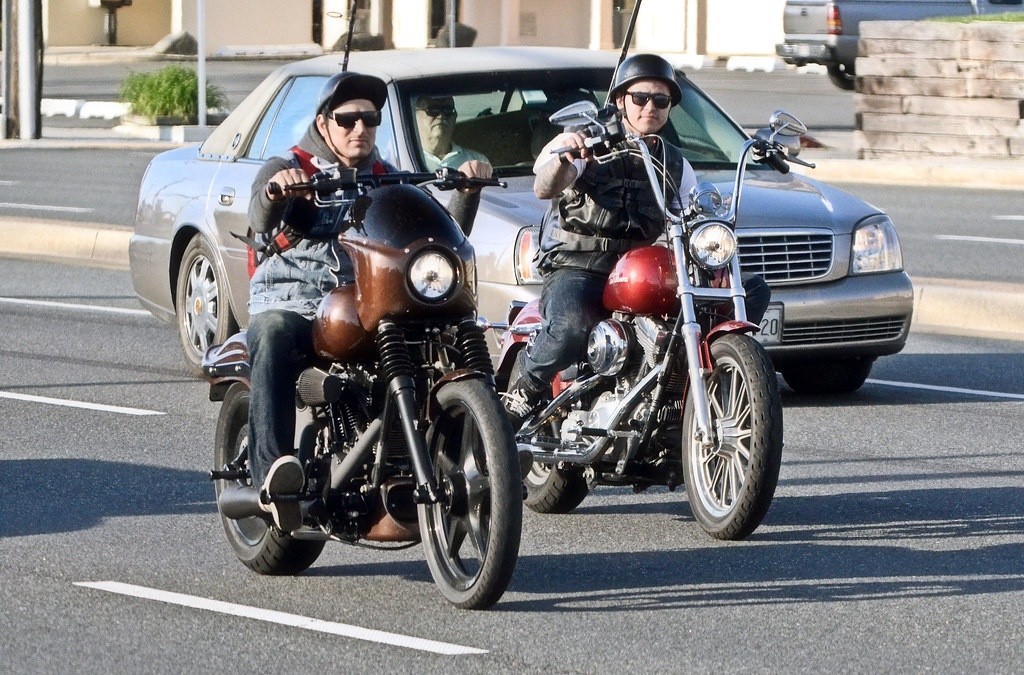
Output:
[198, 166, 537, 612]
[475, 99, 817, 542]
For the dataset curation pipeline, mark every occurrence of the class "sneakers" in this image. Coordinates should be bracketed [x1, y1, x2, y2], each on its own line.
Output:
[263, 456, 305, 530]
[495, 379, 538, 434]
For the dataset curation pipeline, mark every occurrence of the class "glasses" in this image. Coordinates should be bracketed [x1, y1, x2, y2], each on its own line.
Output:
[414, 106, 457, 118]
[324, 111, 381, 127]
[624, 91, 671, 110]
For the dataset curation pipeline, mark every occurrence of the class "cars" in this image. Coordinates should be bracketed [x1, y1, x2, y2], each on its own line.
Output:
[127, 40, 918, 396]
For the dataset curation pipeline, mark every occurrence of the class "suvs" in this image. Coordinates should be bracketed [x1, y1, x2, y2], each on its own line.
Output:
[772, 0, 1019, 92]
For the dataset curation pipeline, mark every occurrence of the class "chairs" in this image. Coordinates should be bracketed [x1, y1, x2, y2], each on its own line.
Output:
[530, 90, 599, 161]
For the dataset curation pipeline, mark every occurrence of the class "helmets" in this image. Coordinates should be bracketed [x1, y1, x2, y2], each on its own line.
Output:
[316, 72, 388, 117]
[607, 54, 681, 108]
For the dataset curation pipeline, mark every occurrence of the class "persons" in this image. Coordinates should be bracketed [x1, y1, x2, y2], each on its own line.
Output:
[498, 54, 773, 436]
[248, 72, 492, 530]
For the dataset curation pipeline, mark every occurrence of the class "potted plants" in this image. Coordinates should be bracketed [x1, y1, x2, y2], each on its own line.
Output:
[117, 61, 229, 126]
